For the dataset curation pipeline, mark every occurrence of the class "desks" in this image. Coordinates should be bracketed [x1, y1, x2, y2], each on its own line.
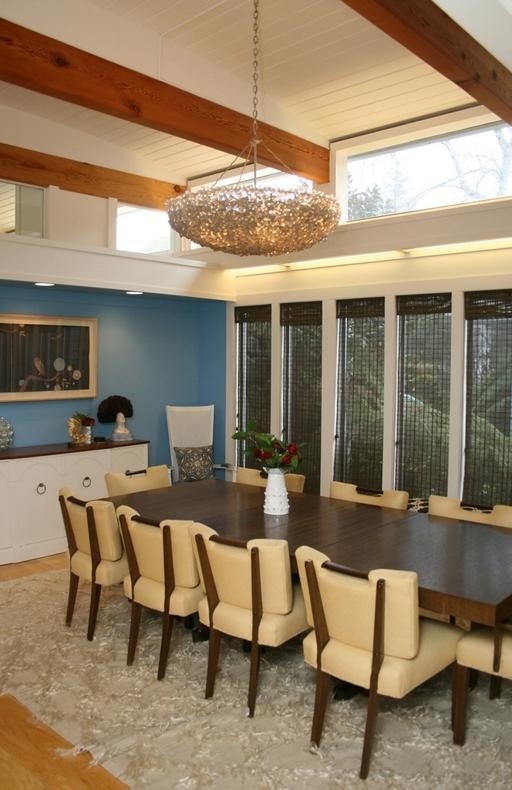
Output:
[90, 478, 512, 632]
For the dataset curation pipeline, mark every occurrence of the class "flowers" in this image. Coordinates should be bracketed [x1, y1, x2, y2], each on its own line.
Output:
[232, 420, 304, 470]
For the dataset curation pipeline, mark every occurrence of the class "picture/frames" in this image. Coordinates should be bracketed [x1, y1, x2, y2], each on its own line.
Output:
[0, 314, 99, 403]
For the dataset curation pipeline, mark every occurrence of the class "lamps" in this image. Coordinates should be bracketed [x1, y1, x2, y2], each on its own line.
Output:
[165, 0, 340, 255]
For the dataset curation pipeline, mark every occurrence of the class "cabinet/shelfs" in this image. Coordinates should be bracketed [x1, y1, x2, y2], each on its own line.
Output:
[0, 438, 150, 570]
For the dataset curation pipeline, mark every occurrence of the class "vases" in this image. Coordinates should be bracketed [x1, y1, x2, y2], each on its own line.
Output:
[263, 466, 290, 515]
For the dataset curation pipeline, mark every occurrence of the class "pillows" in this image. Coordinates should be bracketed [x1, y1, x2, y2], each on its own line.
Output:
[174, 445, 215, 483]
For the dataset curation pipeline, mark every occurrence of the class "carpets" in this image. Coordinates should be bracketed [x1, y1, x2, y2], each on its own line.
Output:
[0, 566, 512, 790]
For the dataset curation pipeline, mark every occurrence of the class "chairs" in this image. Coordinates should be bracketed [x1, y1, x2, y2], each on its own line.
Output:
[429, 495, 512, 528]
[58, 487, 129, 641]
[104, 464, 171, 496]
[165, 405, 215, 482]
[330, 481, 408, 510]
[235, 468, 304, 493]
[191, 524, 308, 718]
[454, 626, 511, 749]
[293, 546, 464, 780]
[116, 505, 201, 680]
